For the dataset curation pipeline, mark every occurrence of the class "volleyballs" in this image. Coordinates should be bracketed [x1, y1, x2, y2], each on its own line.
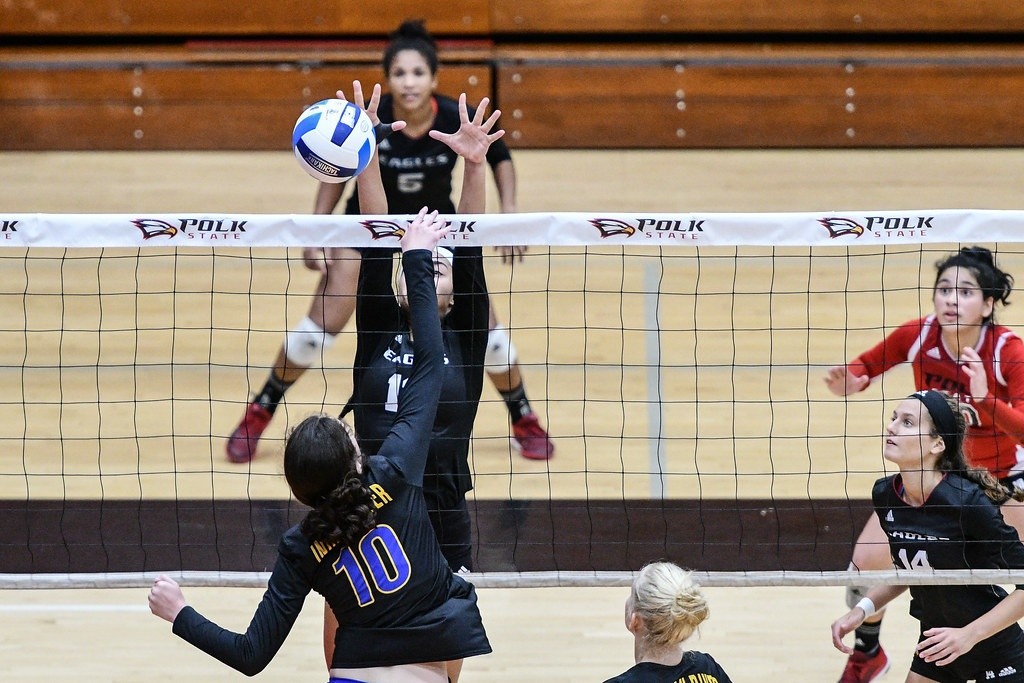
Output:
[288, 94, 380, 189]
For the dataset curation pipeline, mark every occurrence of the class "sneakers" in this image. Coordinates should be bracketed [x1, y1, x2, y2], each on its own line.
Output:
[512, 414, 554, 459]
[838, 645, 891, 683]
[227, 404, 272, 463]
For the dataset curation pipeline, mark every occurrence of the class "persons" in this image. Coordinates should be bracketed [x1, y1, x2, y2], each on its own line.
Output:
[822, 245, 1024, 683]
[147, 18, 554, 683]
[602, 560, 734, 683]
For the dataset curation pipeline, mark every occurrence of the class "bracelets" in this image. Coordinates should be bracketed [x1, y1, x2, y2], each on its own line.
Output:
[855, 597, 876, 621]
